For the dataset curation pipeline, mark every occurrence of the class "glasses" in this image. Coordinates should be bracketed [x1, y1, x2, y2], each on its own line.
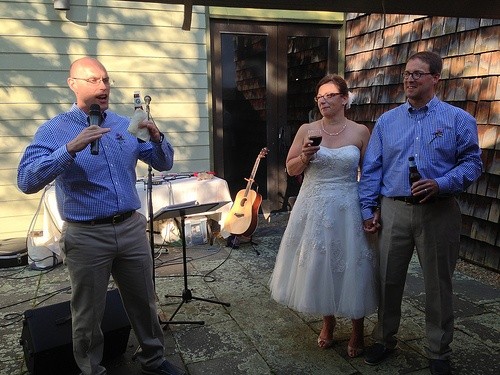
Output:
[402, 71, 432, 80]
[73, 77, 110, 83]
[314, 93, 342, 103]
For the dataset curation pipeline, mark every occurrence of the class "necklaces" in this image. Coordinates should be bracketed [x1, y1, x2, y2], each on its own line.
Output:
[321, 118, 347, 136]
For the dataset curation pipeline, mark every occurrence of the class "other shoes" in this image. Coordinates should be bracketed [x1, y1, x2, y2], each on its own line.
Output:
[430, 358, 451, 375]
[364, 336, 397, 365]
[141, 362, 186, 375]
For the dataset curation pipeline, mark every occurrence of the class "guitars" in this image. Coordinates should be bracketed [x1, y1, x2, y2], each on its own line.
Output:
[225, 146, 271, 237]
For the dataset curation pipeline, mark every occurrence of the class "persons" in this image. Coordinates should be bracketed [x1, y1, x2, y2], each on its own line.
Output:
[357, 51, 484, 375]
[16, 58, 188, 375]
[267, 74, 381, 357]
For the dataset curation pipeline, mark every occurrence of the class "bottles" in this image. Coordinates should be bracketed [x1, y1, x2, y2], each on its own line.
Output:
[134, 91, 146, 144]
[408, 157, 427, 201]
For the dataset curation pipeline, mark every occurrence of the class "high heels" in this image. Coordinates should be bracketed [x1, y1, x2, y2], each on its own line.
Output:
[318, 317, 335, 348]
[348, 325, 364, 358]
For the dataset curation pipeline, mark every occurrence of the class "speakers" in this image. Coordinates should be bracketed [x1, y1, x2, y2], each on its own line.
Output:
[21, 291, 131, 375]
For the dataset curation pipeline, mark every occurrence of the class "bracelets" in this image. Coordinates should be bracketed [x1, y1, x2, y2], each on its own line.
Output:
[160, 134, 163, 143]
[301, 154, 308, 166]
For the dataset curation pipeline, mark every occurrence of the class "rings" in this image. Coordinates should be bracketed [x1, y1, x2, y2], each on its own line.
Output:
[425, 189, 428, 193]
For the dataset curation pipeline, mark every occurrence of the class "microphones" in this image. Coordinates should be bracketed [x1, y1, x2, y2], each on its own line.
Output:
[89, 104, 101, 156]
[144, 96, 151, 112]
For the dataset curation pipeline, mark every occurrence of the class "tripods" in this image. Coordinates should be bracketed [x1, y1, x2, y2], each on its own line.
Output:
[227, 177, 260, 255]
[131, 165, 231, 360]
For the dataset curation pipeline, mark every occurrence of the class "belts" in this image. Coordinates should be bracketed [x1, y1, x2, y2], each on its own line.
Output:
[389, 196, 412, 205]
[67, 209, 137, 224]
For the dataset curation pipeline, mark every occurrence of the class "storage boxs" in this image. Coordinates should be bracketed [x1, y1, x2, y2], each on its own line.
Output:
[177, 215, 207, 243]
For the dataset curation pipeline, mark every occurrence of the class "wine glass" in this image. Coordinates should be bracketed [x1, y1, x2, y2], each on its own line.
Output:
[308, 129, 323, 163]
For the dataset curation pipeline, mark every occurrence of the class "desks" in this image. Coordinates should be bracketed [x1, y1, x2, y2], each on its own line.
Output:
[124, 173, 233, 245]
